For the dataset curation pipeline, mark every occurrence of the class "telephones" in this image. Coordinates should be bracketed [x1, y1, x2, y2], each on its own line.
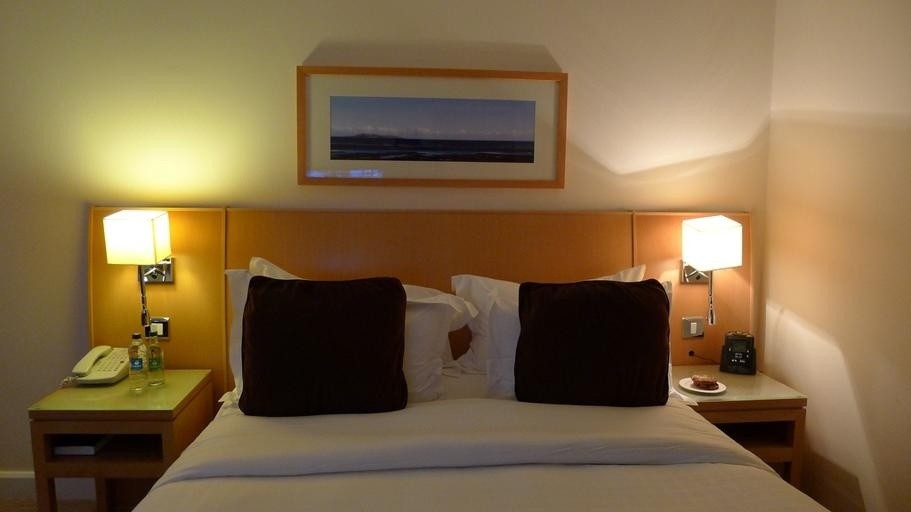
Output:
[59, 345, 129, 389]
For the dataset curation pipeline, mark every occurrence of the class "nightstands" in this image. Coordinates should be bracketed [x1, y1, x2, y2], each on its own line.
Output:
[671, 362, 808, 490]
[26, 368, 212, 512]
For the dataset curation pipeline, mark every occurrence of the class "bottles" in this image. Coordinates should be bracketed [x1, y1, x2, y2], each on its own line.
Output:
[128, 333, 147, 391]
[147, 330, 165, 385]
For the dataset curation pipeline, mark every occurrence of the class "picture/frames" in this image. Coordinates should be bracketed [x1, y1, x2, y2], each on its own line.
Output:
[293, 64, 566, 191]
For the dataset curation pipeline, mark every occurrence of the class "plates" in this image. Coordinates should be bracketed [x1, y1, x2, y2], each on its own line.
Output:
[678, 378, 727, 394]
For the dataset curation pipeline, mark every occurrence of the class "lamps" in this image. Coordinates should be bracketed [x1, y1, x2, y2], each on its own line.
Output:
[102, 208, 174, 340]
[679, 215, 742, 325]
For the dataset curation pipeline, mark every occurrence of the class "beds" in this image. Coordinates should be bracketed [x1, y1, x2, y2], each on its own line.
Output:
[82, 207, 837, 512]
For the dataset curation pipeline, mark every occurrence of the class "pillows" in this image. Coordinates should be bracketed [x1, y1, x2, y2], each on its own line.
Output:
[237, 275, 409, 419]
[217, 255, 476, 403]
[514, 277, 670, 409]
[448, 269, 660, 382]
